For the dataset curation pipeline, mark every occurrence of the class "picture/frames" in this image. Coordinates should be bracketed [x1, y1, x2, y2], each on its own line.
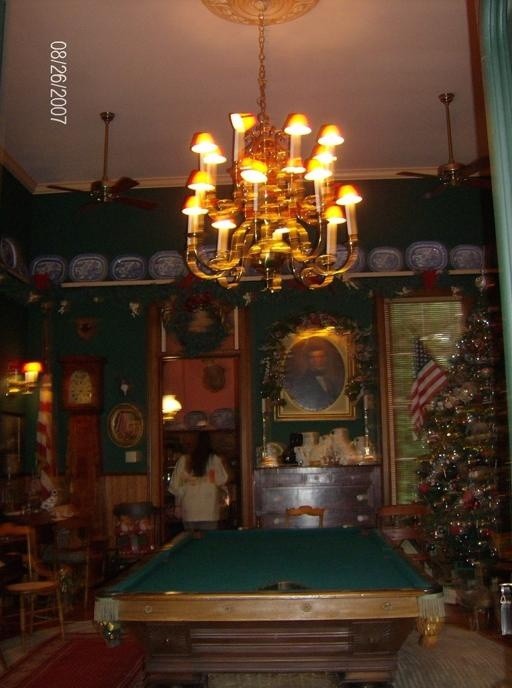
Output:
[259, 311, 371, 421]
[106, 402, 144, 448]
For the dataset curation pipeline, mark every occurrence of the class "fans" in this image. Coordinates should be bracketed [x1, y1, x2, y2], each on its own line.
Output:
[47, 110, 154, 211]
[396, 92, 492, 203]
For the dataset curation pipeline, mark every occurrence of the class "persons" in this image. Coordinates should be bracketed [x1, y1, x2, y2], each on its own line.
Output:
[167, 433, 229, 531]
[291, 345, 339, 413]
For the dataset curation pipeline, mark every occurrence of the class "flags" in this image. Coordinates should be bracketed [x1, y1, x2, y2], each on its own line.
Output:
[408, 338, 453, 448]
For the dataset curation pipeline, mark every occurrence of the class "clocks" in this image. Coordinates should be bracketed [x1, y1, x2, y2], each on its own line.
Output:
[59, 354, 105, 412]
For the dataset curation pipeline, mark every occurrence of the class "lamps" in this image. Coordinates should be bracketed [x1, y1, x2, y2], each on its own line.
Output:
[4, 360, 42, 396]
[162, 393, 182, 423]
[179, 2, 363, 295]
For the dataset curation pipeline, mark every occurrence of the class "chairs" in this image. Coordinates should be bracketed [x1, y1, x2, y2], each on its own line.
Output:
[284, 505, 325, 529]
[377, 502, 428, 564]
[0, 522, 67, 648]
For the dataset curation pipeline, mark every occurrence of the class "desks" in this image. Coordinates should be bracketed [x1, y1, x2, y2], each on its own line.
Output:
[92, 523, 447, 687]
